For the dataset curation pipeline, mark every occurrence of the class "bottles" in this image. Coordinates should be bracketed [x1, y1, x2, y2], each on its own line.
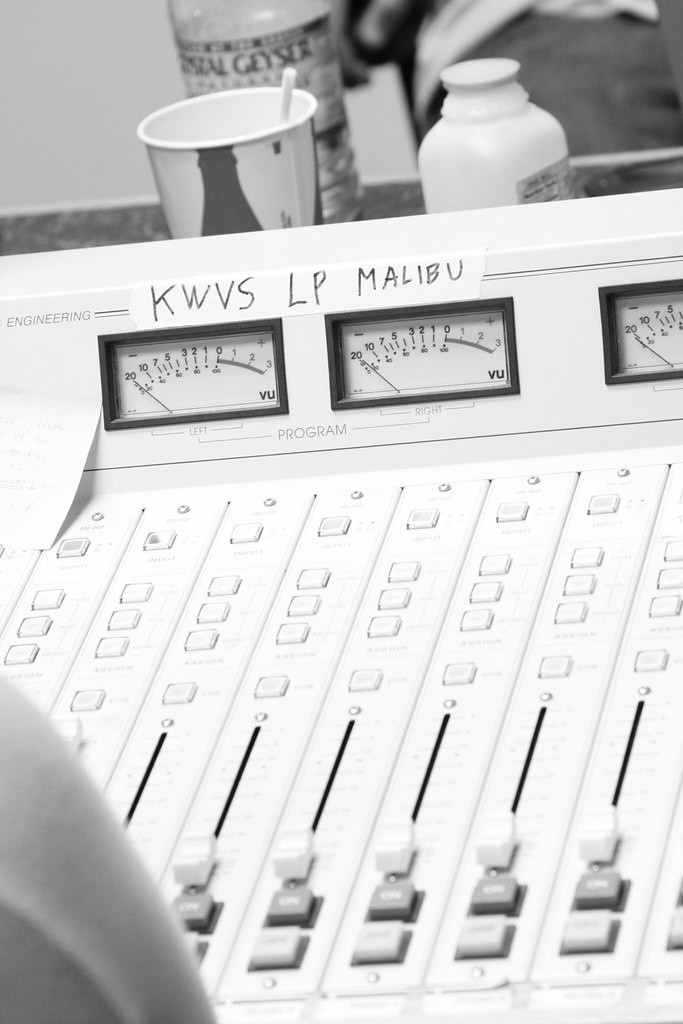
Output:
[160, 0, 364, 228]
[420, 59, 569, 215]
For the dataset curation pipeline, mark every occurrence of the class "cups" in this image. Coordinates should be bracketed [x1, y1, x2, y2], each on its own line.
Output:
[137, 87, 323, 241]
